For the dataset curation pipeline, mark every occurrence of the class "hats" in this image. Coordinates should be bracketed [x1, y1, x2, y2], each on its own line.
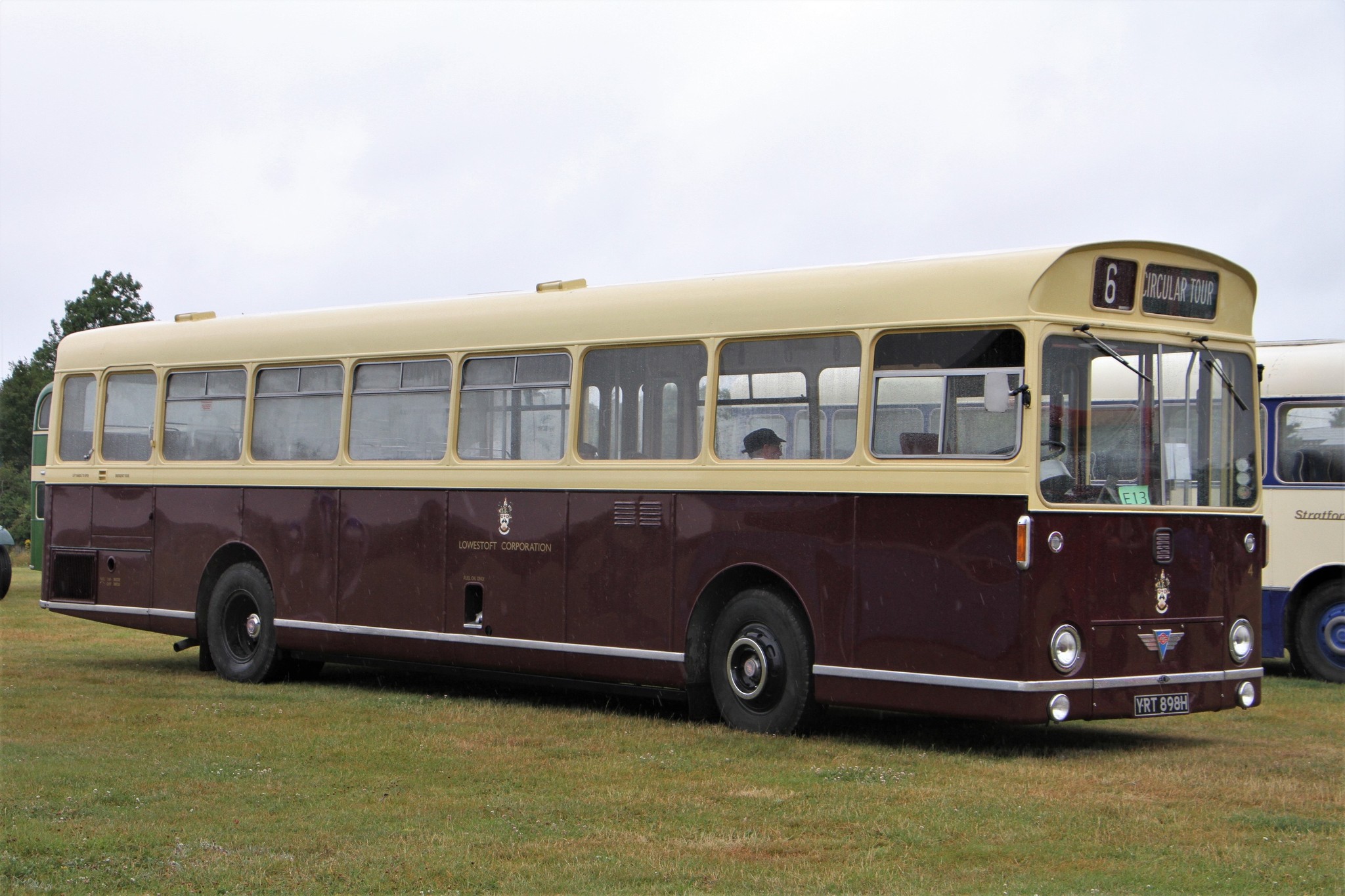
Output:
[742, 428, 786, 454]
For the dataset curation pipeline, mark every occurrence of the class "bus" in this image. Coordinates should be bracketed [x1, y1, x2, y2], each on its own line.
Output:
[39, 237, 1270, 737]
[580, 336, 1345, 684]
[29, 379, 53, 570]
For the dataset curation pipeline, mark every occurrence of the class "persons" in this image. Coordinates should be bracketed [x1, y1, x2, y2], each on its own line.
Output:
[741, 429, 786, 462]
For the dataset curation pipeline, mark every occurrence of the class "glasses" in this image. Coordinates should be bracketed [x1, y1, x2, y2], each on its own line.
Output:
[760, 444, 782, 453]
